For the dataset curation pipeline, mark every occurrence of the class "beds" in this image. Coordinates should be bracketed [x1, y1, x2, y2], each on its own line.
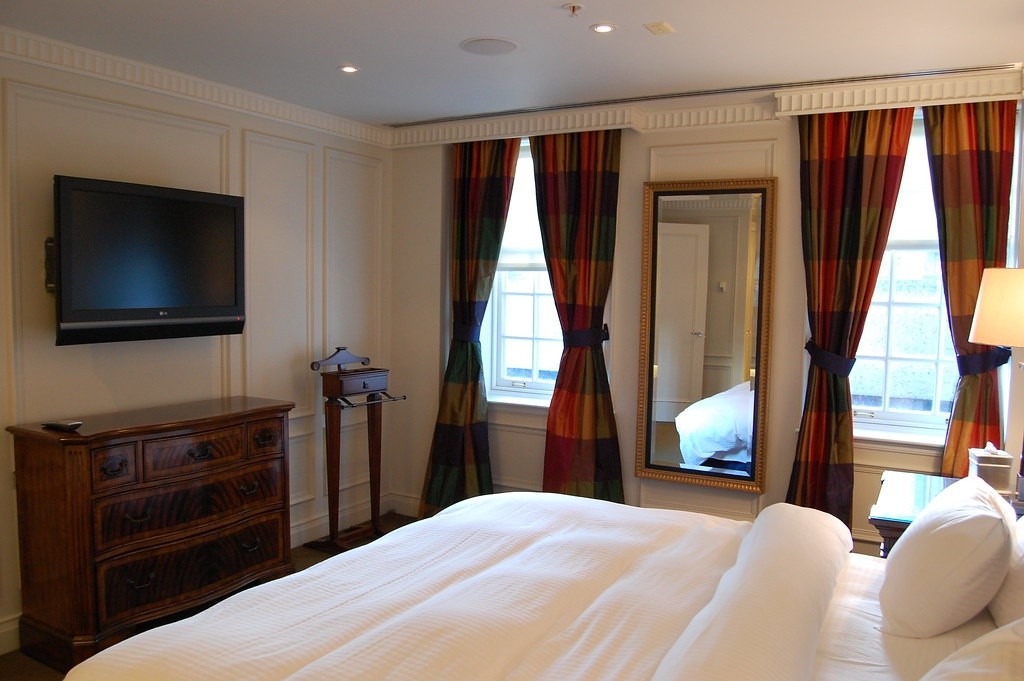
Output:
[59, 489, 1023, 681]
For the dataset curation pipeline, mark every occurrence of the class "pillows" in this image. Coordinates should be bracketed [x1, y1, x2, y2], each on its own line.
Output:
[879, 476, 1017, 639]
[918, 617, 1023, 681]
[985, 515, 1024, 628]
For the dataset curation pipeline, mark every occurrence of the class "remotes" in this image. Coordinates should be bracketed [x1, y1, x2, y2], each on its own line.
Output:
[41, 419, 82, 432]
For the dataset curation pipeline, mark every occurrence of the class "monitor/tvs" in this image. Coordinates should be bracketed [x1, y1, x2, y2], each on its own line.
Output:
[54, 174, 246, 346]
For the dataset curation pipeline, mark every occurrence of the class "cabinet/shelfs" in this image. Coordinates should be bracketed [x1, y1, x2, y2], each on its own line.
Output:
[3, 394, 295, 672]
[303, 346, 408, 553]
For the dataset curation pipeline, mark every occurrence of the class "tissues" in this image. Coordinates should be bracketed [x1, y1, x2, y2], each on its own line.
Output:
[967, 440, 1014, 497]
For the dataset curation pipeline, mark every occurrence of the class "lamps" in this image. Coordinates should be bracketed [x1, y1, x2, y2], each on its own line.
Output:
[966, 266, 1023, 516]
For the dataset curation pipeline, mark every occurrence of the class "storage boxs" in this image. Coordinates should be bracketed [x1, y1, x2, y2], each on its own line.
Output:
[968, 447, 1014, 491]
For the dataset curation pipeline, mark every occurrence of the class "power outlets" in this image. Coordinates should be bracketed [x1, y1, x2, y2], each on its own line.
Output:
[643, 20, 675, 35]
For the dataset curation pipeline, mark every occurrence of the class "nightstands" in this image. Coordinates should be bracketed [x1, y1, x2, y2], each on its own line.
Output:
[868, 470, 1019, 559]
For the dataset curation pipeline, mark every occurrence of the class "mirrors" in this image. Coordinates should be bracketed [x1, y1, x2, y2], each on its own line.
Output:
[634, 176, 776, 493]
